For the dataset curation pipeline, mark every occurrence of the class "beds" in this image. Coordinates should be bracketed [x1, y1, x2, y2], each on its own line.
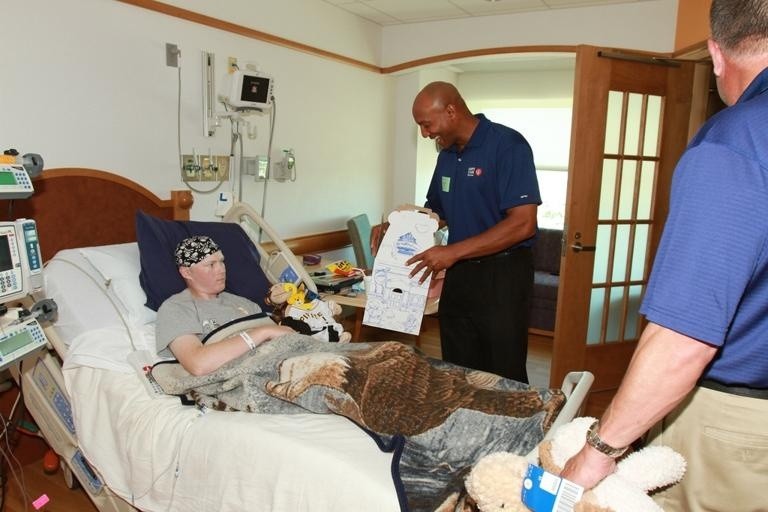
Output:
[1, 168, 594, 510]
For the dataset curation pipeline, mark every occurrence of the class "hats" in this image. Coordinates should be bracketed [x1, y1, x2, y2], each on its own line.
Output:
[174, 235, 222, 268]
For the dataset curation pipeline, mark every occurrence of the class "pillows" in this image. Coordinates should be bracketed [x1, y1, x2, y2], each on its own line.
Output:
[135, 206, 276, 313]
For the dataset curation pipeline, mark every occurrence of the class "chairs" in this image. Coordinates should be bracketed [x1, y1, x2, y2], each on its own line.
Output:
[347, 213, 427, 349]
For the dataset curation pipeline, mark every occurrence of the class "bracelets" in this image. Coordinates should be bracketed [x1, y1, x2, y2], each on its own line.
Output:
[237, 329, 259, 352]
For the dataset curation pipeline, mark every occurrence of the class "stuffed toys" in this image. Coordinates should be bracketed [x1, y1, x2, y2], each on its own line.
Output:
[464, 418, 688, 511]
[264, 281, 352, 347]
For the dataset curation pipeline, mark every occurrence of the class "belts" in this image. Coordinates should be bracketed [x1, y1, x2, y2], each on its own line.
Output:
[698, 374, 737, 398]
[459, 248, 524, 264]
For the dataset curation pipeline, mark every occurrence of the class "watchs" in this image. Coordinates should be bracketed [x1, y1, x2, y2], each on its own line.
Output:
[586, 421, 627, 458]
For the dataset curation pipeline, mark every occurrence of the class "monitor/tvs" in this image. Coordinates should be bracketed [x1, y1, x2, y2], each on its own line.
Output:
[230, 68, 275, 109]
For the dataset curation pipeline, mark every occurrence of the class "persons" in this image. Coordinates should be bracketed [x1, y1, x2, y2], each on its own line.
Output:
[156, 236, 352, 389]
[559, 1, 767, 510]
[369, 81, 543, 383]
